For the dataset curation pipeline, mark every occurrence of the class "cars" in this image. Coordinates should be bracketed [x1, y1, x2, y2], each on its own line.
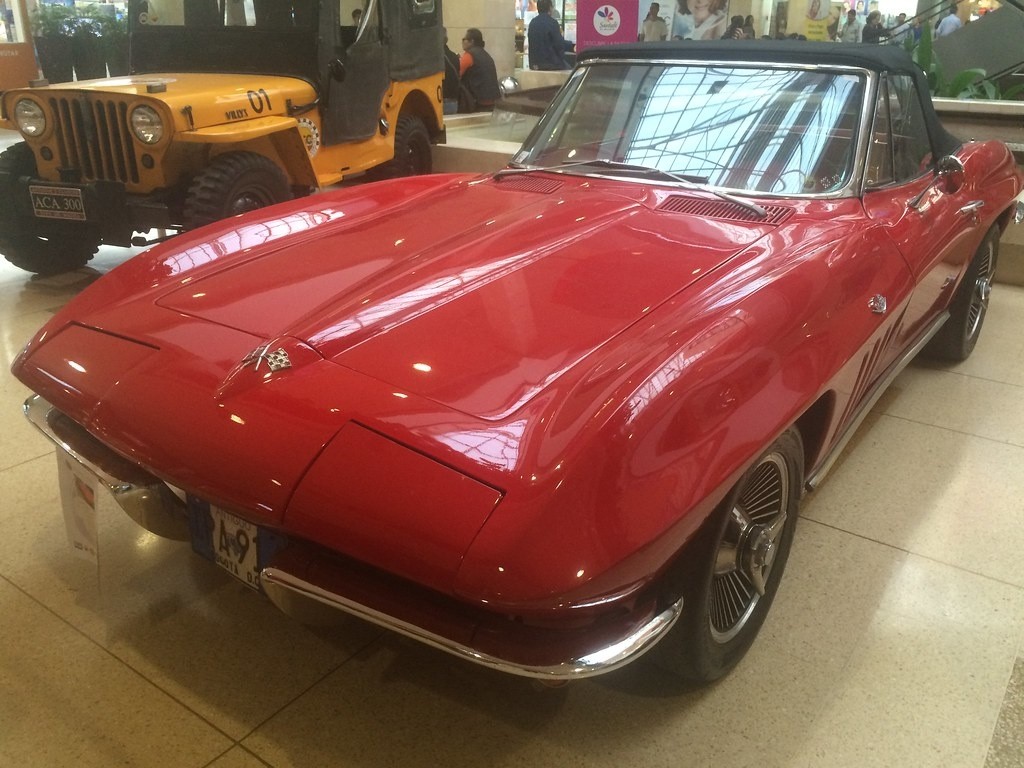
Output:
[12, 42, 1023, 684]
[0, 0, 448, 271]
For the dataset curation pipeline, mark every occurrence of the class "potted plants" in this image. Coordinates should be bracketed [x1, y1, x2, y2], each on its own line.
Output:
[28, 1, 129, 84]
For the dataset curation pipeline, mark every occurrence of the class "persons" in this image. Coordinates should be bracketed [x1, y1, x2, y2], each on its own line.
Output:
[460, 28, 501, 115]
[892, 13, 922, 44]
[861, 10, 891, 43]
[869, 1, 877, 12]
[857, 1, 863, 11]
[670, 0, 727, 41]
[810, 0, 820, 19]
[638, 3, 668, 42]
[443, 27, 460, 115]
[826, 6, 840, 26]
[936, 5, 961, 40]
[843, 2, 850, 12]
[837, 9, 859, 42]
[721, 14, 807, 40]
[529, 0, 572, 70]
[352, 9, 360, 25]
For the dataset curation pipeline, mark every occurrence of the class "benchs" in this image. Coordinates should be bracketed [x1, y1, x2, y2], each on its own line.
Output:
[522, 68, 573, 88]
[688, 124, 912, 187]
